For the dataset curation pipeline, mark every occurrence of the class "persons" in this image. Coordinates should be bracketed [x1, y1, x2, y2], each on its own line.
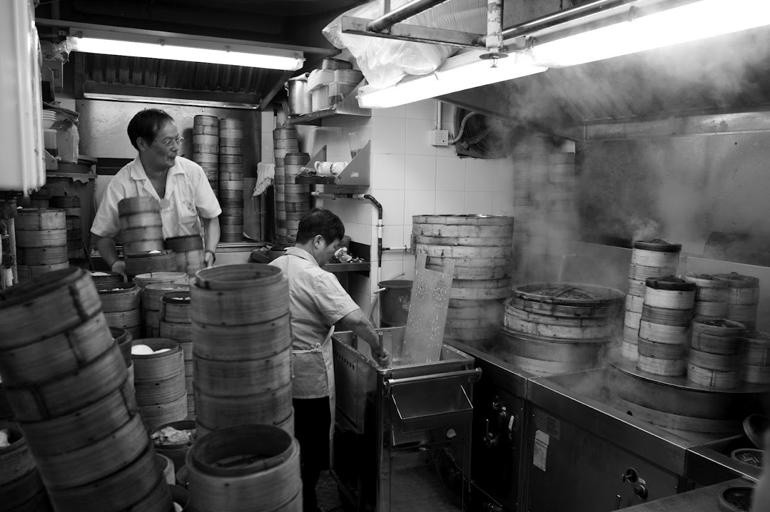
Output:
[264, 205, 394, 511]
[86, 107, 224, 285]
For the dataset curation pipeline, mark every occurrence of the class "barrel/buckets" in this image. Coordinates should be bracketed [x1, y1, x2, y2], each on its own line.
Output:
[283, 68, 364, 115]
[30, 154, 98, 248]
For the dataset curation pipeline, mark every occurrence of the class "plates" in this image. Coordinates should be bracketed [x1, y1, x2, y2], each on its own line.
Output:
[42, 109, 57, 130]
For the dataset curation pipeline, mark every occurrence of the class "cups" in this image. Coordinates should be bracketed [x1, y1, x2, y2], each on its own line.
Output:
[330, 162, 349, 173]
[314, 160, 335, 176]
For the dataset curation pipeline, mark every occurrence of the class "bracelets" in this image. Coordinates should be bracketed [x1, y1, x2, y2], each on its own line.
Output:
[204, 249, 217, 265]
[109, 259, 119, 268]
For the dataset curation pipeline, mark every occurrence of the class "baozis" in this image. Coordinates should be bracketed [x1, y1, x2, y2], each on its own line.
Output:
[130, 343, 172, 356]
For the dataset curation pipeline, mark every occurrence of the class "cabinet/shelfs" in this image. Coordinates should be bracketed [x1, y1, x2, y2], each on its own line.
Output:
[294, 139, 371, 185]
[285, 76, 372, 120]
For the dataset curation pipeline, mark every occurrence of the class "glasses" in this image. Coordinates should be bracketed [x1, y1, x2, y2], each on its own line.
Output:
[142, 138, 185, 148]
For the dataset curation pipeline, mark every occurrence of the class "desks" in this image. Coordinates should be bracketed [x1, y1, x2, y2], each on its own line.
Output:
[251, 240, 368, 289]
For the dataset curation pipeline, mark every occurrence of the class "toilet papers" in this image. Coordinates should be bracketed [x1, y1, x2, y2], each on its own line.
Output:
[317, 161, 348, 176]
